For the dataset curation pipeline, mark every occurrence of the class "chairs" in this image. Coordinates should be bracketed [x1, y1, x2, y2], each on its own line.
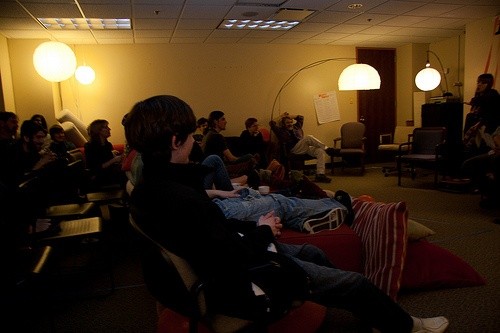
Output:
[327, 122, 366, 176]
[127, 211, 373, 333]
[14, 148, 134, 303]
[280, 142, 316, 168]
[376, 126, 448, 186]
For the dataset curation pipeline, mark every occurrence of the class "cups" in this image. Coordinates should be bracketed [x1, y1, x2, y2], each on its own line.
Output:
[258, 186, 270, 194]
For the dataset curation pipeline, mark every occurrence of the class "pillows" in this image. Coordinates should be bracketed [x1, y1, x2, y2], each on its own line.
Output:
[400, 241, 487, 290]
[349, 196, 408, 302]
[408, 219, 437, 242]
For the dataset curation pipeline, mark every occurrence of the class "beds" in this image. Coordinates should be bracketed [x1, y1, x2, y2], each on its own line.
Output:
[278, 225, 361, 272]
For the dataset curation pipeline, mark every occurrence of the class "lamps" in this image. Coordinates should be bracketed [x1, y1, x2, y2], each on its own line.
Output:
[32, 41, 77, 111]
[271, 58, 381, 121]
[414, 50, 452, 96]
[75, 66, 96, 85]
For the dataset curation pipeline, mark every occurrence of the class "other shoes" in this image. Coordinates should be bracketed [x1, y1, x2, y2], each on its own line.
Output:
[411, 316, 449, 333]
[326, 147, 341, 157]
[259, 169, 272, 179]
[302, 208, 346, 235]
[315, 173, 331, 183]
[335, 189, 355, 227]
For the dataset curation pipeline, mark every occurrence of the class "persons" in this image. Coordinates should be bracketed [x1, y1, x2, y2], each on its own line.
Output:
[0, 94, 452, 332]
[461, 72, 500, 149]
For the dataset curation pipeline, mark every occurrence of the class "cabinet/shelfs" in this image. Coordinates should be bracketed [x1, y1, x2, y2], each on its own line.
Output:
[422, 102, 463, 144]
